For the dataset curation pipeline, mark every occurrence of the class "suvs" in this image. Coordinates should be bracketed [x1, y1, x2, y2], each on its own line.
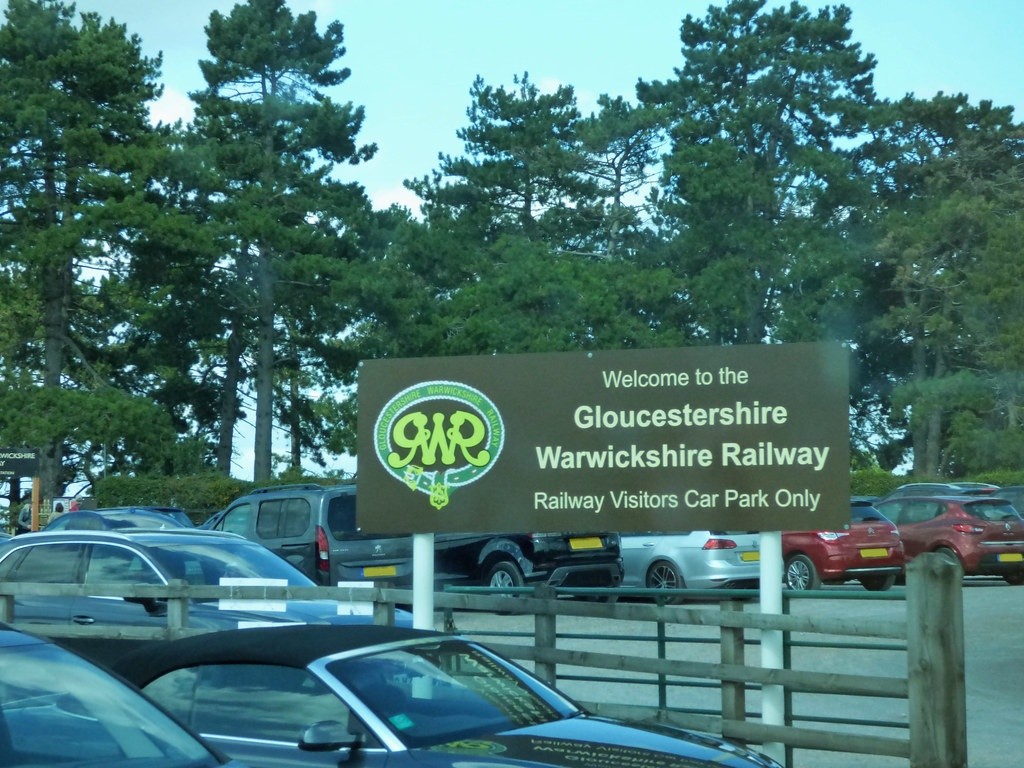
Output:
[876, 481, 999, 496]
[210, 482, 413, 589]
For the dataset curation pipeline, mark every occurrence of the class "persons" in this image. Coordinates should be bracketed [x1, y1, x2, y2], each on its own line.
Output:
[47, 503, 63, 524]
[18, 499, 32, 534]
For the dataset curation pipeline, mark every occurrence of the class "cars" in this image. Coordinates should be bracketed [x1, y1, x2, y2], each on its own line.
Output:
[196, 512, 223, 531]
[43, 508, 185, 531]
[435, 532, 623, 603]
[0, 530, 412, 626]
[149, 506, 195, 527]
[876, 495, 1024, 586]
[620, 531, 787, 604]
[0, 623, 253, 768]
[781, 497, 904, 590]
[991, 486, 1024, 518]
[0, 529, 14, 544]
[111, 626, 783, 768]
